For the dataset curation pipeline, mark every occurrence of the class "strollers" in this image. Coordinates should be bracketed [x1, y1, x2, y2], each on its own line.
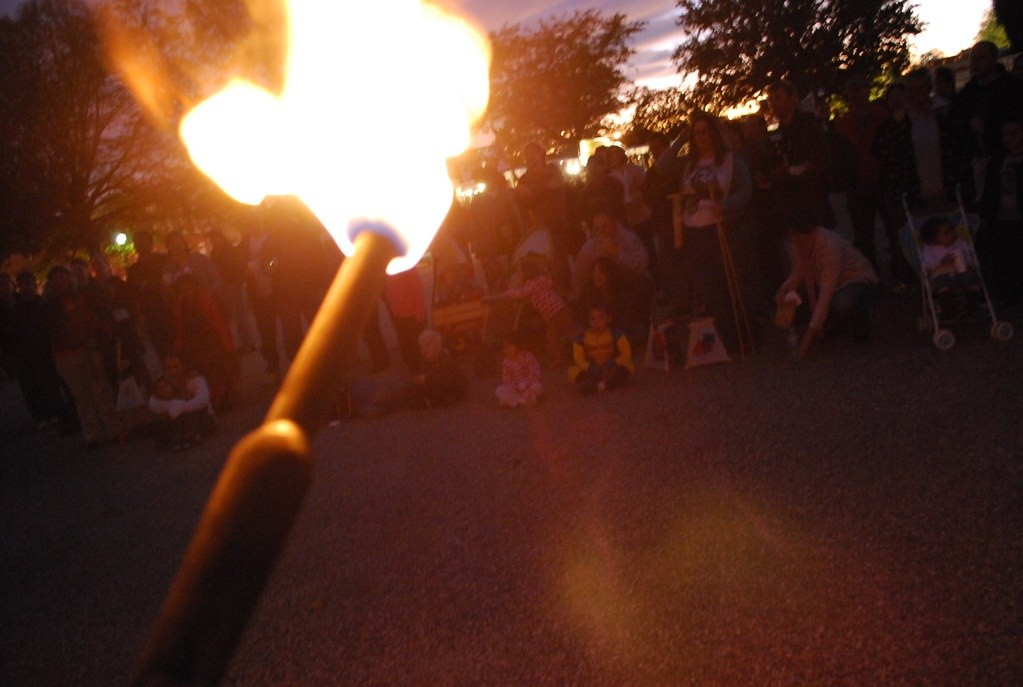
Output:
[903, 181, 1015, 351]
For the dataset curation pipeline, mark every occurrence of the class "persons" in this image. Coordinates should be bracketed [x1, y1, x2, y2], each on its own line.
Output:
[773, 209, 875, 360]
[573, 304, 634, 392]
[922, 217, 985, 316]
[0, 43, 1023, 451]
[495, 333, 544, 408]
[413, 330, 469, 408]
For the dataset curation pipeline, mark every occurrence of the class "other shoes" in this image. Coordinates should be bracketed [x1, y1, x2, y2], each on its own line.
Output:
[818, 321, 849, 342]
[856, 319, 871, 344]
